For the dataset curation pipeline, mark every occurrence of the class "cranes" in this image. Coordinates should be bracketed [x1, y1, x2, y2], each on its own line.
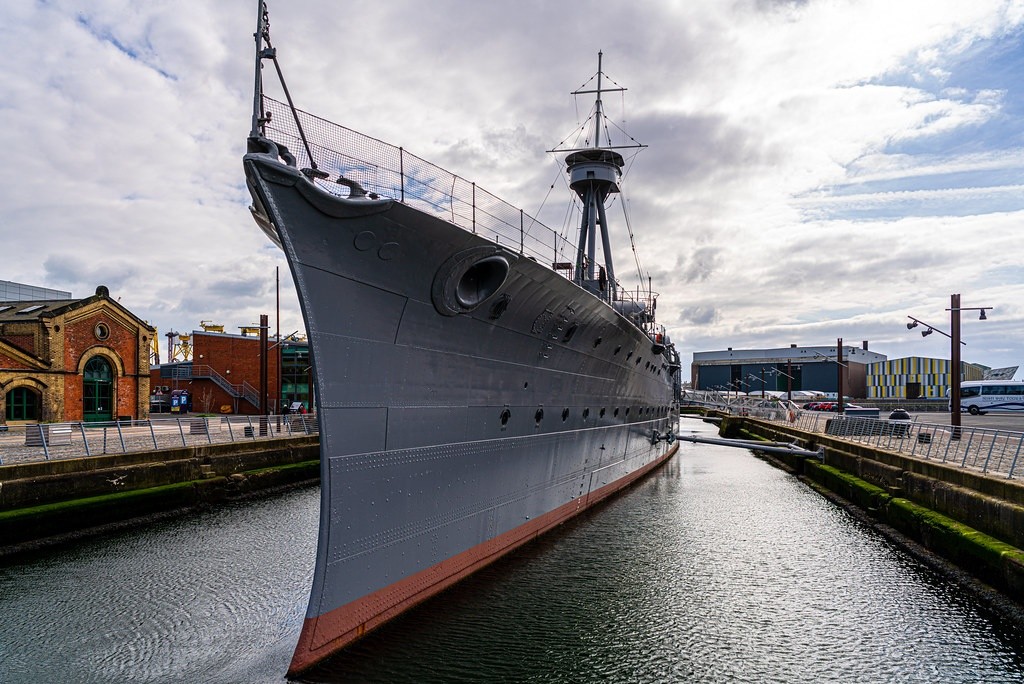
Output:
[165, 328, 179, 363]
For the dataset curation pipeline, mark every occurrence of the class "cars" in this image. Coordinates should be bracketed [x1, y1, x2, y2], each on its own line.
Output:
[779, 391, 815, 400]
[809, 402, 860, 412]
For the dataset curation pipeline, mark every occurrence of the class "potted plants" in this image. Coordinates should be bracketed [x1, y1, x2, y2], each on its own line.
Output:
[190, 414, 221, 435]
[26, 418, 73, 446]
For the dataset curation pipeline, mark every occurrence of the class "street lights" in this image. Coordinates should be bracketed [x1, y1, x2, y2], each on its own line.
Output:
[749, 367, 767, 398]
[736, 374, 751, 396]
[771, 359, 794, 403]
[907, 294, 993, 441]
[814, 338, 848, 412]
[238, 314, 299, 436]
[707, 381, 739, 398]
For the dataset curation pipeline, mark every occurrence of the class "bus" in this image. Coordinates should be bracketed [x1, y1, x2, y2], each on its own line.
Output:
[946, 380, 1024, 415]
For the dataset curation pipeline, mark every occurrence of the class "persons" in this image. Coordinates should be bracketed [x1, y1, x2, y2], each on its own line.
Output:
[297, 404, 307, 414]
[281, 404, 291, 425]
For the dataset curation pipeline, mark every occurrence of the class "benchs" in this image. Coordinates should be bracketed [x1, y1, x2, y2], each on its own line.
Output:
[992, 390, 998, 394]
[1000, 391, 1005, 395]
[985, 390, 991, 394]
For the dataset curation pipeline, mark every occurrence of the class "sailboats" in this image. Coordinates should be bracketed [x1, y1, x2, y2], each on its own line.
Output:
[242, 0, 681, 679]
